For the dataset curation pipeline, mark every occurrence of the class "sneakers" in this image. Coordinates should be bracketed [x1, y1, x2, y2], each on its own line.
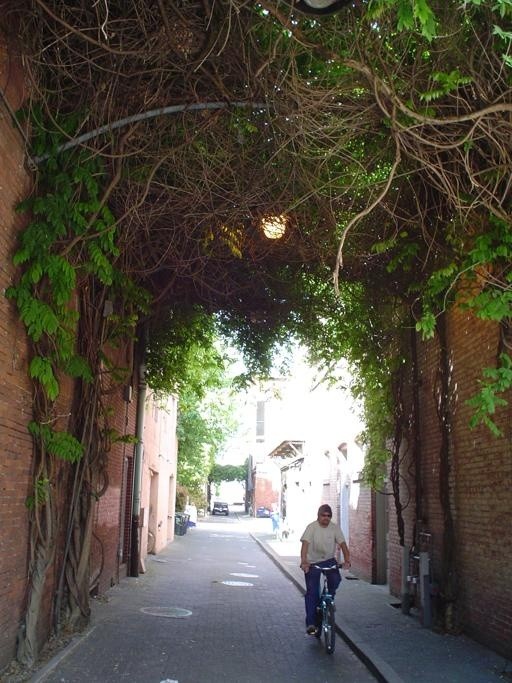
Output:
[306, 625, 316, 636]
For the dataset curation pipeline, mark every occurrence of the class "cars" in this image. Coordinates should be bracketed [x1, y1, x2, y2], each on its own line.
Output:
[256, 506, 272, 518]
[212, 501, 229, 516]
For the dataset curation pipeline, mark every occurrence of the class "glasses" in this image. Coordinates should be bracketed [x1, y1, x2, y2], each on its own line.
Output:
[320, 514, 331, 518]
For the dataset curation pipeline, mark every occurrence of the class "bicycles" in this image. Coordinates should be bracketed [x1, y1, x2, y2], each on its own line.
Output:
[300, 562, 352, 654]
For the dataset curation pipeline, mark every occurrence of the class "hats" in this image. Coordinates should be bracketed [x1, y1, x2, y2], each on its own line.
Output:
[318, 505, 333, 516]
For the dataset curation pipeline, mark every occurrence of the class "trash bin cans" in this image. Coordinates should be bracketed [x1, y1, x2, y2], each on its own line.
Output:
[174, 512, 190, 536]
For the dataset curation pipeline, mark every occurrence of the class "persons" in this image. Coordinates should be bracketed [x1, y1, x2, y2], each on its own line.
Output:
[300, 504, 351, 635]
